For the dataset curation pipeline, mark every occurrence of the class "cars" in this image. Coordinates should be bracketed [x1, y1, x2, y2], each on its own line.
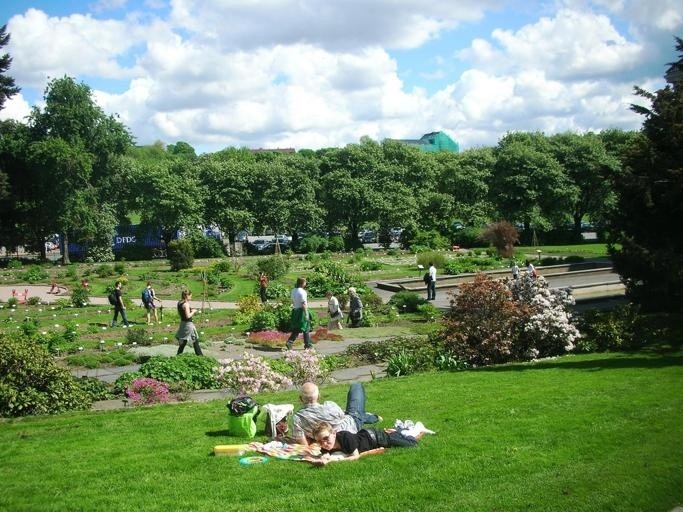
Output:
[252, 234, 288, 251]
[358, 231, 375, 243]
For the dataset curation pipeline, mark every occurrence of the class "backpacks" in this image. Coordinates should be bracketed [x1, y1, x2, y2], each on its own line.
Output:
[108, 292, 117, 305]
[142, 288, 152, 303]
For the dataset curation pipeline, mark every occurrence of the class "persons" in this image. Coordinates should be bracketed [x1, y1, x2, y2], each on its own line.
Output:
[141, 281, 163, 325]
[46, 275, 60, 294]
[325, 291, 343, 330]
[285, 277, 316, 351]
[424, 262, 437, 301]
[275, 382, 436, 466]
[10, 288, 30, 303]
[511, 261, 520, 280]
[525, 258, 537, 279]
[258, 271, 270, 302]
[174, 289, 204, 357]
[347, 287, 362, 326]
[107, 280, 134, 328]
[80, 278, 90, 289]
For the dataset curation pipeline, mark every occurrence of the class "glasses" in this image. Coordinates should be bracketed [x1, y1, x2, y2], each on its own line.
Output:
[317, 434, 330, 444]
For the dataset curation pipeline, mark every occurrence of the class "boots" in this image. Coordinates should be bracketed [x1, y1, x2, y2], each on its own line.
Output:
[286, 342, 313, 350]
[427, 298, 435, 300]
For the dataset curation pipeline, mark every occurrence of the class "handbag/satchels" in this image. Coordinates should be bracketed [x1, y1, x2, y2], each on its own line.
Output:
[349, 311, 360, 320]
[226, 397, 261, 439]
